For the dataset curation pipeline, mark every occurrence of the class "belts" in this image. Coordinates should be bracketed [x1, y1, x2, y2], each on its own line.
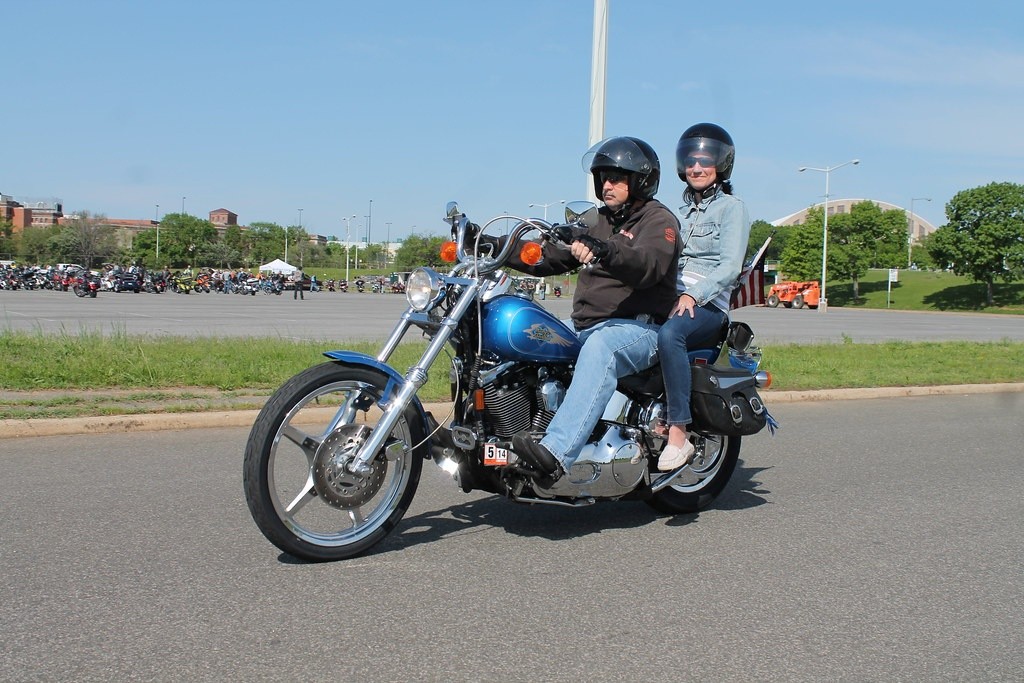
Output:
[618, 312, 667, 326]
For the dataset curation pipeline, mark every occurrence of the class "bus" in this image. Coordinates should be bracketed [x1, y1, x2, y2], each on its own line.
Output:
[511, 276, 546, 295]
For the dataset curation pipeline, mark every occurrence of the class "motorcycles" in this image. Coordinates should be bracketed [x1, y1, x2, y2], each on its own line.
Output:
[242, 199, 779, 561]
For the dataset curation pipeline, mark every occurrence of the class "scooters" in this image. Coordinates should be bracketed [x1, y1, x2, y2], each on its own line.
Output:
[0, 259, 408, 299]
[553, 286, 562, 298]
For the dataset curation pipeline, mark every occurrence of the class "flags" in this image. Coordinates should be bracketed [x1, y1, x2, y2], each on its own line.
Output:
[728, 240, 766, 312]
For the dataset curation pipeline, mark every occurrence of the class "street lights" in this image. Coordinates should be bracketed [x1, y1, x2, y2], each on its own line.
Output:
[528, 200, 565, 221]
[297, 208, 304, 267]
[341, 215, 355, 286]
[907, 197, 933, 266]
[798, 159, 860, 313]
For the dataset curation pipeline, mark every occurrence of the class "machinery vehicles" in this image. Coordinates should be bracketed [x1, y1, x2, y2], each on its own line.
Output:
[764, 280, 820, 309]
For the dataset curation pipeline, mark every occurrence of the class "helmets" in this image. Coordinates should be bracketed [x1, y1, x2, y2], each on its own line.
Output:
[677, 123, 735, 182]
[590, 137, 661, 202]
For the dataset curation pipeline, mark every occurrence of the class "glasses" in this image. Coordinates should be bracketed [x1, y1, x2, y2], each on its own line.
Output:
[599, 170, 625, 184]
[684, 156, 717, 168]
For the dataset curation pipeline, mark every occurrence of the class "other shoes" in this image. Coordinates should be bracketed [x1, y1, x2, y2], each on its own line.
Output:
[513, 432, 563, 491]
[657, 439, 694, 471]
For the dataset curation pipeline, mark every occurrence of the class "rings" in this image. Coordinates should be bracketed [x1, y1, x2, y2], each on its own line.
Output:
[681, 304, 685, 307]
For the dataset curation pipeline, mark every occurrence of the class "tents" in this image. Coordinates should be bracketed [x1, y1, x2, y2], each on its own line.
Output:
[258, 258, 297, 277]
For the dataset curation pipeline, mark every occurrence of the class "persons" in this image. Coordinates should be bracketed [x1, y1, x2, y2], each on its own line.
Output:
[232, 268, 255, 281]
[659, 123, 751, 471]
[378, 274, 386, 294]
[162, 266, 171, 292]
[10, 262, 16, 269]
[45, 264, 53, 271]
[270, 270, 285, 289]
[30, 263, 41, 270]
[222, 269, 232, 294]
[309, 273, 319, 292]
[122, 263, 143, 281]
[455, 135, 682, 484]
[519, 277, 545, 300]
[184, 265, 194, 279]
[67, 263, 74, 270]
[294, 267, 304, 300]
[0, 262, 4, 268]
